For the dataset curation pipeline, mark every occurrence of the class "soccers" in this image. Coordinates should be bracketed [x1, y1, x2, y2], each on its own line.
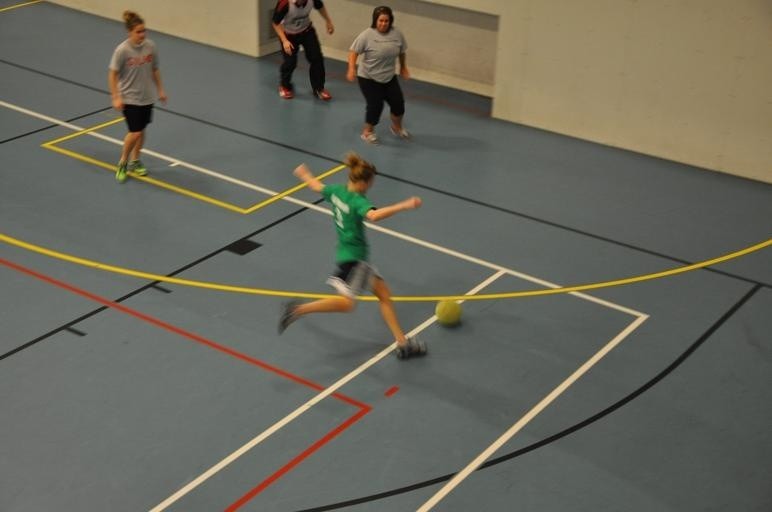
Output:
[435, 301, 463, 326]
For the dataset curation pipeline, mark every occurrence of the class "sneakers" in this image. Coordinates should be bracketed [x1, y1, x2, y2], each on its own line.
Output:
[313, 88, 332, 100]
[361, 130, 379, 145]
[114, 160, 127, 183]
[127, 159, 147, 176]
[278, 82, 294, 98]
[278, 297, 304, 335]
[395, 335, 426, 359]
[390, 125, 411, 139]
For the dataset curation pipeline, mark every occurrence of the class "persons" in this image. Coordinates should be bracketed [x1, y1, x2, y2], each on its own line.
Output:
[108, 9, 168, 185]
[270, 1, 335, 102]
[276, 151, 428, 362]
[345, 5, 415, 147]
